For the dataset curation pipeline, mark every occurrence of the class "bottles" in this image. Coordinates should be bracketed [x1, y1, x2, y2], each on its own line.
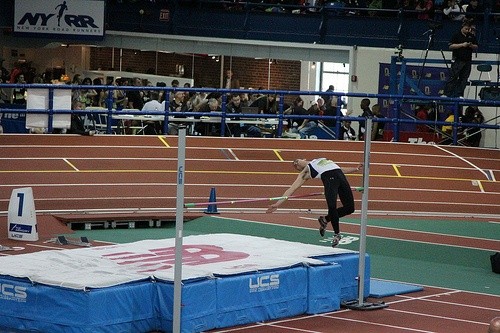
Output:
[231, 108, 236, 119]
[154, 107, 158, 111]
[259, 110, 266, 122]
[275, 111, 280, 124]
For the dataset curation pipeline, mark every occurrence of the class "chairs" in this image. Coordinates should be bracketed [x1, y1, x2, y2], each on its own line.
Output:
[77, 90, 351, 137]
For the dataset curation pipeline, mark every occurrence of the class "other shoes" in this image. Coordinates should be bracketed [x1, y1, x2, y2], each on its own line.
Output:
[332, 234, 341, 247]
[318, 216, 327, 236]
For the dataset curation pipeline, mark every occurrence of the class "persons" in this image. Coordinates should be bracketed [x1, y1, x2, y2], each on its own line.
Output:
[200, 0, 500, 20]
[444, 20, 478, 98]
[1, 67, 484, 147]
[267, 157, 356, 247]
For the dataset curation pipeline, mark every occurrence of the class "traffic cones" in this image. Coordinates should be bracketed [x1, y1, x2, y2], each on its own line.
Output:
[203, 187, 220, 214]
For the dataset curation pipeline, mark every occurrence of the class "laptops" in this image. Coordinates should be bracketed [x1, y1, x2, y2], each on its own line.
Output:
[240, 107, 259, 119]
